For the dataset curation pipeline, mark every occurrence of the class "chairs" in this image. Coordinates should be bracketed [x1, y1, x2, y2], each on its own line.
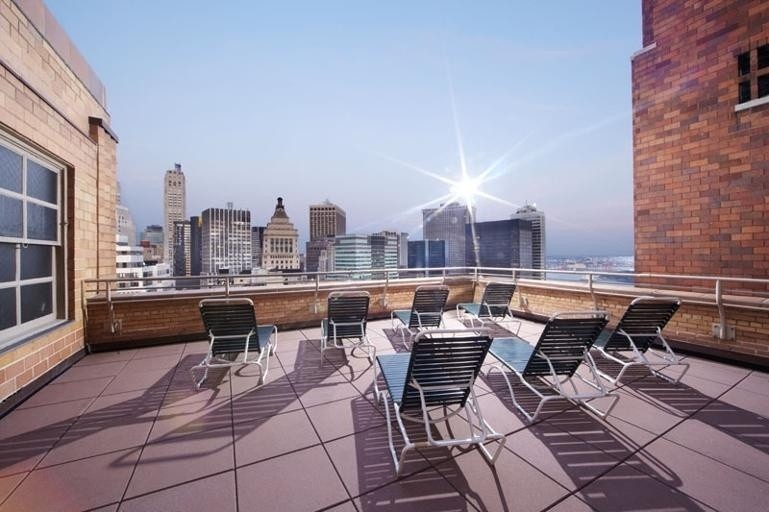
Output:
[475, 310, 620, 424]
[390, 285, 456, 353]
[189, 296, 278, 392]
[372, 325, 507, 478]
[320, 289, 377, 368]
[457, 278, 522, 339]
[586, 295, 690, 387]
